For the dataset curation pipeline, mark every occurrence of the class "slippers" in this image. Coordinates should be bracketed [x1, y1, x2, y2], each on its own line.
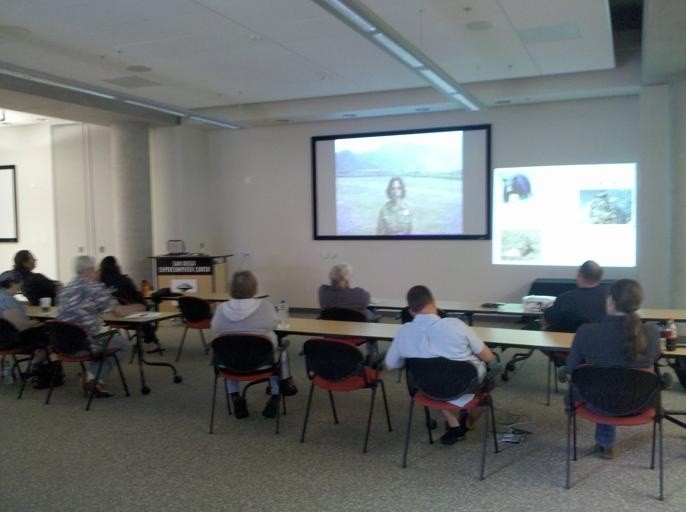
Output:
[146, 344, 166, 353]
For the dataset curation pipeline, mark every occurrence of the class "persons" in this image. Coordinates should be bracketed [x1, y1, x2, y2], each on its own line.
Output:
[542, 260, 612, 382]
[382, 285, 503, 445]
[55, 256, 131, 398]
[210, 271, 287, 420]
[11, 249, 60, 307]
[0, 271, 54, 377]
[375, 176, 415, 234]
[96, 256, 161, 354]
[563, 278, 662, 460]
[318, 263, 384, 369]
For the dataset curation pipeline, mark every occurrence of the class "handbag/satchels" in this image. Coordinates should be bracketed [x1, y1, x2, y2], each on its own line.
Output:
[32, 360, 64, 389]
[267, 376, 298, 396]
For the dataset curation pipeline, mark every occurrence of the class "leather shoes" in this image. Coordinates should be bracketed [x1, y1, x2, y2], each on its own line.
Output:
[263, 395, 279, 418]
[441, 413, 468, 444]
[232, 395, 246, 419]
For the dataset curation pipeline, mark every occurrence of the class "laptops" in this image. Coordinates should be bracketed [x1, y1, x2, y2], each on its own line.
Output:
[168, 240, 188, 256]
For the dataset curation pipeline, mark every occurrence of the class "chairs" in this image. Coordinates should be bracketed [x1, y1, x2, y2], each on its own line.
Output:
[561, 364, 669, 501]
[299, 306, 388, 371]
[500, 318, 569, 410]
[291, 337, 394, 455]
[208, 332, 290, 435]
[175, 296, 214, 364]
[400, 355, 498, 482]
[43, 319, 133, 412]
[0, 316, 58, 404]
[398, 305, 501, 372]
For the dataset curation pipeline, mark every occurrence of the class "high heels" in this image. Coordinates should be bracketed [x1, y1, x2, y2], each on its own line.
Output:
[78, 373, 114, 397]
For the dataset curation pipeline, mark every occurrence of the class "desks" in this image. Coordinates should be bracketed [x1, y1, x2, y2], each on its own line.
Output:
[143, 293, 269, 331]
[529, 277, 616, 299]
[10, 304, 184, 396]
[275, 316, 685, 430]
[146, 253, 234, 293]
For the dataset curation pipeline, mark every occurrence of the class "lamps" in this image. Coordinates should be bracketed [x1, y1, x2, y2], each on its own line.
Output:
[0, 62, 251, 133]
[315, 0, 487, 114]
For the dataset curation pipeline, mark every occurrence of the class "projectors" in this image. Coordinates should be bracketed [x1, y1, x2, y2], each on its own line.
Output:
[521, 294, 559, 314]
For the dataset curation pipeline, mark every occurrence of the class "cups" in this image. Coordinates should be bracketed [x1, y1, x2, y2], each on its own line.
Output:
[39, 297, 52, 313]
[659, 338, 666, 350]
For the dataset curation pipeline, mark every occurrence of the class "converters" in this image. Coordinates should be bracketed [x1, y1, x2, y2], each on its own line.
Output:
[500, 433, 522, 444]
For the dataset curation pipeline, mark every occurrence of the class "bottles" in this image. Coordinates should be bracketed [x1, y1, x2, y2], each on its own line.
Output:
[666, 315, 678, 351]
[140, 279, 149, 296]
[277, 300, 290, 329]
